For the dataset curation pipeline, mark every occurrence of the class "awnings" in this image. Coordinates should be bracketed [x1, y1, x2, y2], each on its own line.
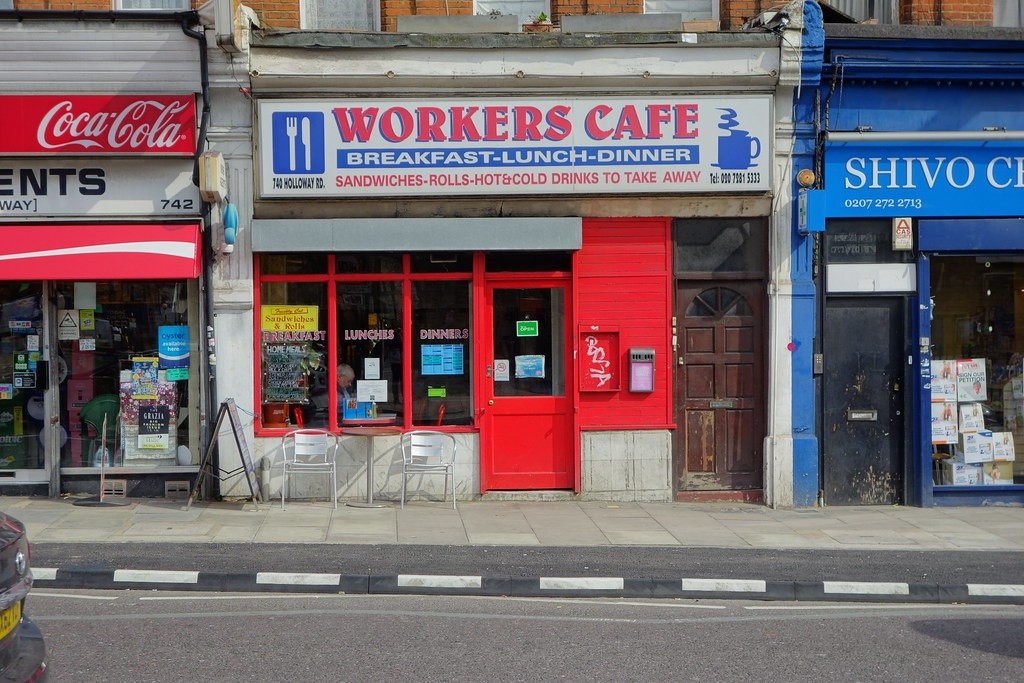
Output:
[0, 217, 199, 280]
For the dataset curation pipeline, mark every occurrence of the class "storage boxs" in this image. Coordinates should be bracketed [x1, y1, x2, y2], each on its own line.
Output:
[931, 359, 1015, 487]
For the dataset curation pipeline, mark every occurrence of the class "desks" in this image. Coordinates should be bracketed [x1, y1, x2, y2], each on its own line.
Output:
[340, 428, 400, 508]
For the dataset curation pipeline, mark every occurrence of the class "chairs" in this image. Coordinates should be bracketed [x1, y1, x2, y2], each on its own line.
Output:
[400, 430, 456, 511]
[281, 429, 339, 511]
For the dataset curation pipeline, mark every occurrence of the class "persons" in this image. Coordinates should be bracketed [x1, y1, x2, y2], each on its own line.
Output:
[337, 364, 355, 418]
[971, 381, 984, 399]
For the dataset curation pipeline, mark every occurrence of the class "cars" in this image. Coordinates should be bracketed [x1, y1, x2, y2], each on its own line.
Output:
[0, 512, 46, 683]
